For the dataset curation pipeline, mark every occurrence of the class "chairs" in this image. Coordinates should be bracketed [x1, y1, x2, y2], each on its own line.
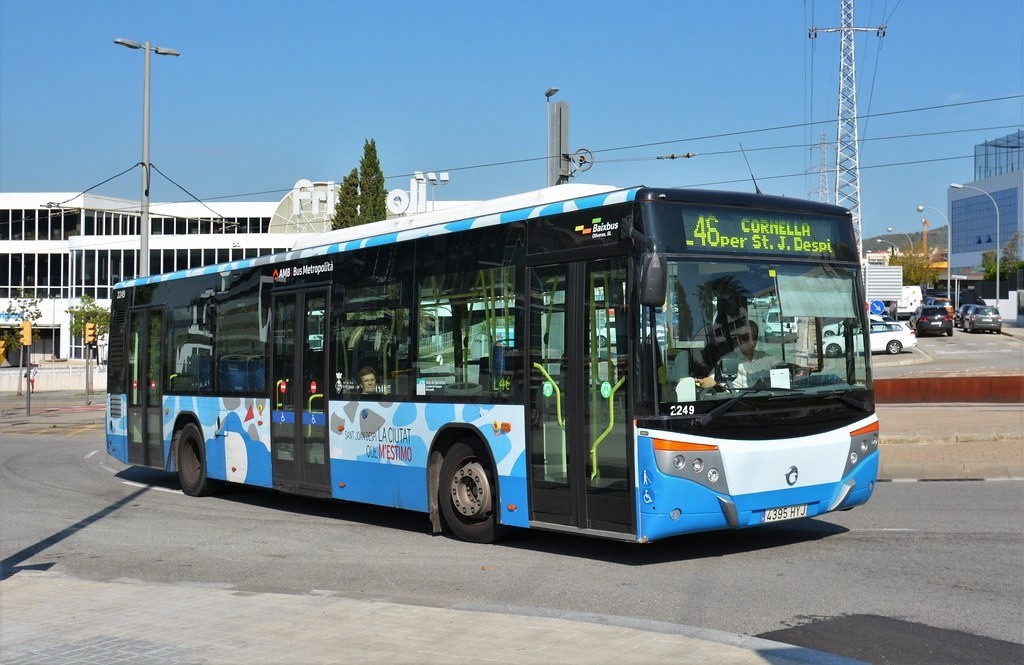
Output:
[196, 352, 264, 392]
[493, 339, 518, 371]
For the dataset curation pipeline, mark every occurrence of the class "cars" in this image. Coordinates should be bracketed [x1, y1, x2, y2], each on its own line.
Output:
[938, 292, 987, 305]
[588, 318, 666, 350]
[954, 303, 978, 328]
[963, 305, 1003, 335]
[925, 297, 953, 308]
[812, 321, 919, 358]
[821, 315, 889, 338]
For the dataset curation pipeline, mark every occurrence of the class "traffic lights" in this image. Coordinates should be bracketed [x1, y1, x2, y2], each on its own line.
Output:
[84, 321, 97, 345]
[0, 340, 6, 365]
[19, 321, 32, 346]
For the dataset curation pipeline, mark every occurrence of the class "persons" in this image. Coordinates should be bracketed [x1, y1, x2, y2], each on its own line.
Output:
[688, 320, 807, 396]
[26, 364, 37, 393]
[358, 366, 378, 395]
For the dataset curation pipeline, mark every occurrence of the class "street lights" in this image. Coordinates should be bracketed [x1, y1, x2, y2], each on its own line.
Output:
[887, 227, 914, 254]
[949, 182, 1000, 311]
[876, 239, 896, 249]
[114, 35, 181, 278]
[543, 87, 560, 189]
[916, 204, 951, 301]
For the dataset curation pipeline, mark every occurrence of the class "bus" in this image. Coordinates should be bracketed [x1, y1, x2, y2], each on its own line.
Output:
[305, 310, 326, 351]
[104, 183, 880, 546]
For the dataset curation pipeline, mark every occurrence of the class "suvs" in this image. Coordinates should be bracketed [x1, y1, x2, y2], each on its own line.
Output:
[909, 305, 954, 337]
[761, 306, 801, 343]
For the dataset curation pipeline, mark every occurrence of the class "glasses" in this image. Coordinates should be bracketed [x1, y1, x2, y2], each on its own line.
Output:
[740, 334, 758, 341]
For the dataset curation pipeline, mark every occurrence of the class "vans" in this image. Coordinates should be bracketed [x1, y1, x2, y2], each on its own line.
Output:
[889, 286, 924, 322]
